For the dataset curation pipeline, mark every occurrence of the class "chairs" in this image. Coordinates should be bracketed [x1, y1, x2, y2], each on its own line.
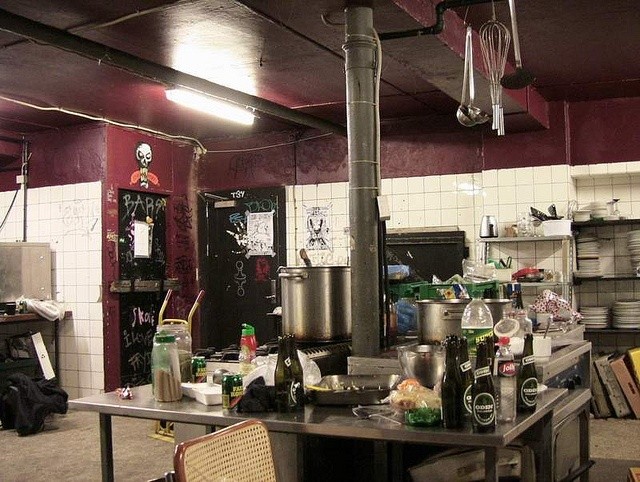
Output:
[175, 419, 281, 481]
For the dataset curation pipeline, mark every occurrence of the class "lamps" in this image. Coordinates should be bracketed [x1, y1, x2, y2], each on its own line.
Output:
[164, 86, 256, 126]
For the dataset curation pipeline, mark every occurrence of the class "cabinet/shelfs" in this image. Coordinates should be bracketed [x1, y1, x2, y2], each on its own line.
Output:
[478, 237, 572, 290]
[0, 311, 71, 385]
[572, 218, 640, 335]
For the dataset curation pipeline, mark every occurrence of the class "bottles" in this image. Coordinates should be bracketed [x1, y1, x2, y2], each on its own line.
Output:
[285, 332, 305, 411]
[470, 340, 495, 434]
[460, 290, 494, 361]
[274, 334, 298, 413]
[516, 333, 538, 414]
[456, 336, 476, 423]
[151, 334, 183, 402]
[493, 336, 517, 422]
[151, 323, 193, 395]
[441, 339, 466, 430]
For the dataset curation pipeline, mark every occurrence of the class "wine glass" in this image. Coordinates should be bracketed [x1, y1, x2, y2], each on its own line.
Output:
[516, 210, 535, 237]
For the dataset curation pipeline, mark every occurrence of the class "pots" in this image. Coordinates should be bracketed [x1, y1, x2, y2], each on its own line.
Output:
[275, 264, 352, 342]
[415, 296, 513, 353]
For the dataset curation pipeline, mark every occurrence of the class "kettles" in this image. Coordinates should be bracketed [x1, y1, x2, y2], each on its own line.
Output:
[479, 214, 499, 238]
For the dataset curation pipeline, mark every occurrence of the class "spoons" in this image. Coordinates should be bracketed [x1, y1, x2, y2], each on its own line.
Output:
[456, 27, 477, 128]
[467, 27, 490, 125]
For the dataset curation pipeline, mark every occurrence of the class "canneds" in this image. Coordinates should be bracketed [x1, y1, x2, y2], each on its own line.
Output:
[192, 356, 207, 383]
[222, 371, 243, 413]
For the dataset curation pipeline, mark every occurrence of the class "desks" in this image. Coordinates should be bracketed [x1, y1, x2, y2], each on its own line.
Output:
[67, 385, 566, 481]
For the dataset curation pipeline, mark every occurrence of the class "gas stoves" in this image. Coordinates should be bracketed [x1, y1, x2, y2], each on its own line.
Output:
[194, 340, 351, 364]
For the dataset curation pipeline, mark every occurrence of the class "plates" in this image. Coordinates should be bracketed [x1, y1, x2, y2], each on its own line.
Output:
[572, 200, 610, 221]
[580, 306, 610, 330]
[572, 232, 602, 278]
[626, 229, 640, 276]
[613, 300, 640, 330]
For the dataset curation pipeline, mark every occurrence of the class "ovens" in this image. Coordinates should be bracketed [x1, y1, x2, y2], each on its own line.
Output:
[545, 354, 597, 482]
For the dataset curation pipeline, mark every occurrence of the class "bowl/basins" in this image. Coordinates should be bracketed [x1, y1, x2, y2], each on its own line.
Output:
[395, 344, 447, 388]
